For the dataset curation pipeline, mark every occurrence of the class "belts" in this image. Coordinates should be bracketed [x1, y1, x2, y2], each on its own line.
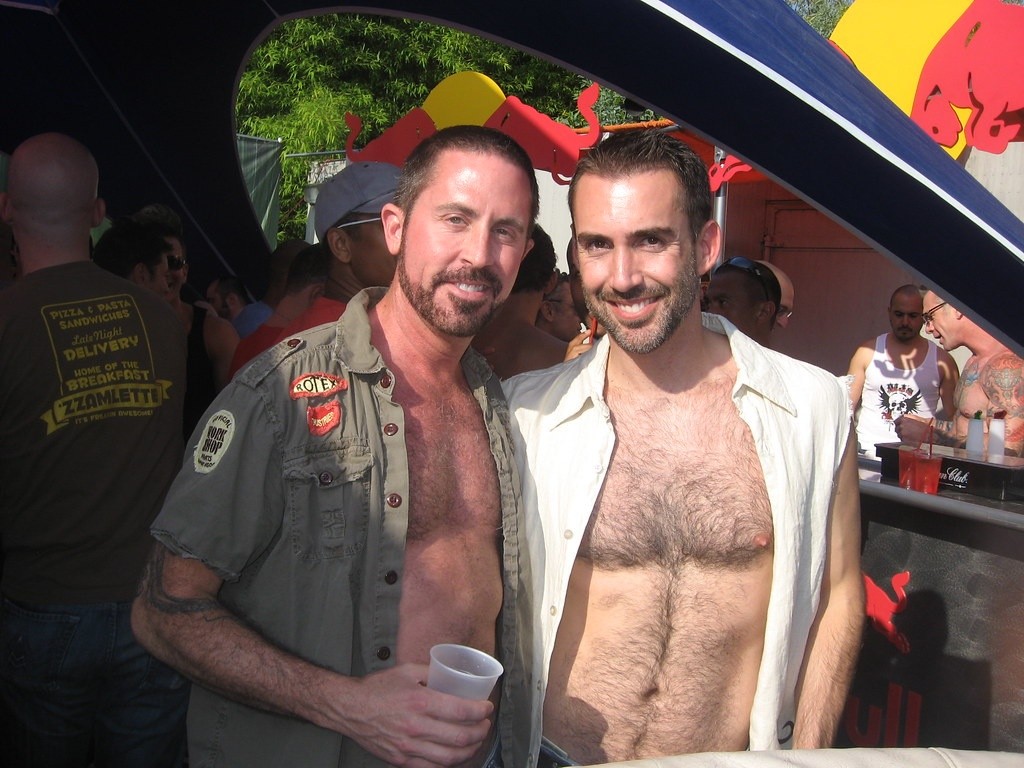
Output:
[498, 740, 568, 768]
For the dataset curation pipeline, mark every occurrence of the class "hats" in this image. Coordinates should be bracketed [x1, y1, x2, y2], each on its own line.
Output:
[313, 159, 404, 247]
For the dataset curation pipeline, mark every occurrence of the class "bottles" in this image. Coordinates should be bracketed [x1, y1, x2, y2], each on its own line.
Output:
[987, 410, 1007, 464]
[966, 410, 983, 459]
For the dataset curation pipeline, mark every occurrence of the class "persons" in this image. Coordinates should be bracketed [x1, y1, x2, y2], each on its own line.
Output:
[0, 119, 1024, 768]
[845, 284, 959, 460]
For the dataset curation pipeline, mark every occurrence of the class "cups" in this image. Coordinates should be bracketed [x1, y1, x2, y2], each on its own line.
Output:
[899, 445, 925, 490]
[426, 644, 503, 725]
[915, 453, 943, 494]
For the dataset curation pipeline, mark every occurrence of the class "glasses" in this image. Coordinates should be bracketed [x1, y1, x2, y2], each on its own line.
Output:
[777, 305, 794, 322]
[714, 255, 771, 303]
[168, 254, 188, 273]
[921, 300, 947, 326]
